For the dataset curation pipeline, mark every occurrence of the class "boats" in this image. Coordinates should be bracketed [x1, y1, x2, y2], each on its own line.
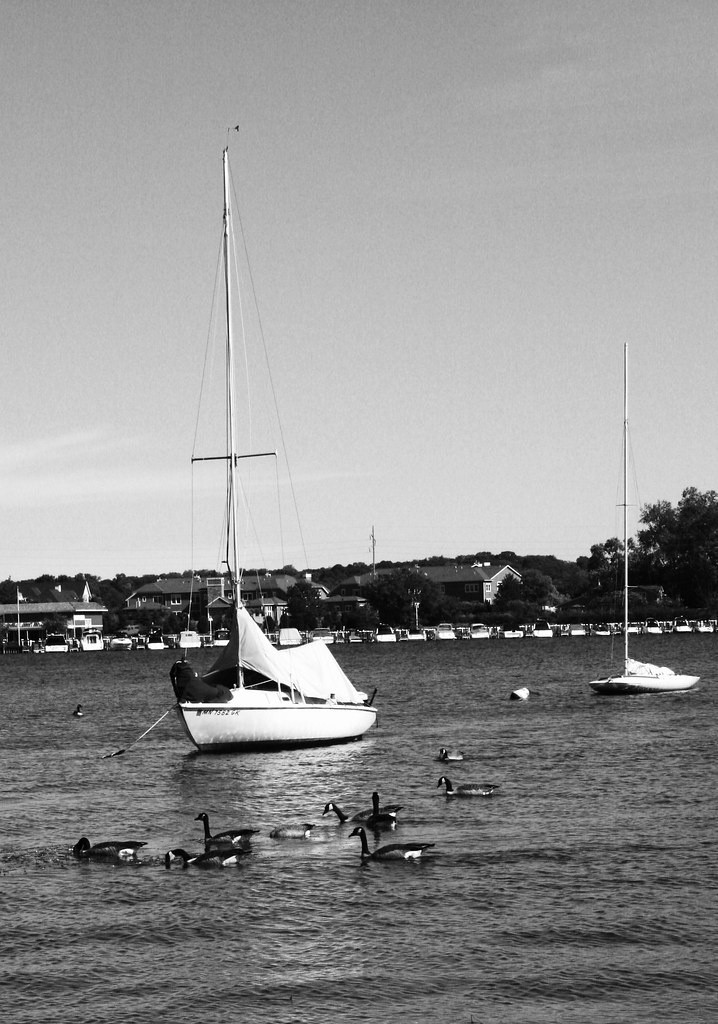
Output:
[77, 628, 105, 652]
[568, 616, 717, 637]
[532, 619, 554, 638]
[216, 628, 232, 648]
[276, 627, 302, 646]
[469, 623, 490, 639]
[177, 630, 201, 649]
[44, 633, 69, 654]
[369, 624, 397, 644]
[308, 627, 335, 646]
[144, 631, 169, 651]
[436, 623, 458, 640]
[110, 631, 134, 652]
[347, 630, 363, 643]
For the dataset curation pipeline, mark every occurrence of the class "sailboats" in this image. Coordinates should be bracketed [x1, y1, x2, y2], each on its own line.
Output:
[587, 344, 706, 694]
[157, 146, 380, 752]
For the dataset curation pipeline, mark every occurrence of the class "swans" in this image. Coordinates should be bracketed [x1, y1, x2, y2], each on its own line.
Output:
[269, 824, 315, 839]
[436, 776, 500, 795]
[73, 704, 84, 716]
[164, 812, 261, 866]
[439, 748, 465, 760]
[73, 837, 148, 857]
[322, 792, 435, 860]
